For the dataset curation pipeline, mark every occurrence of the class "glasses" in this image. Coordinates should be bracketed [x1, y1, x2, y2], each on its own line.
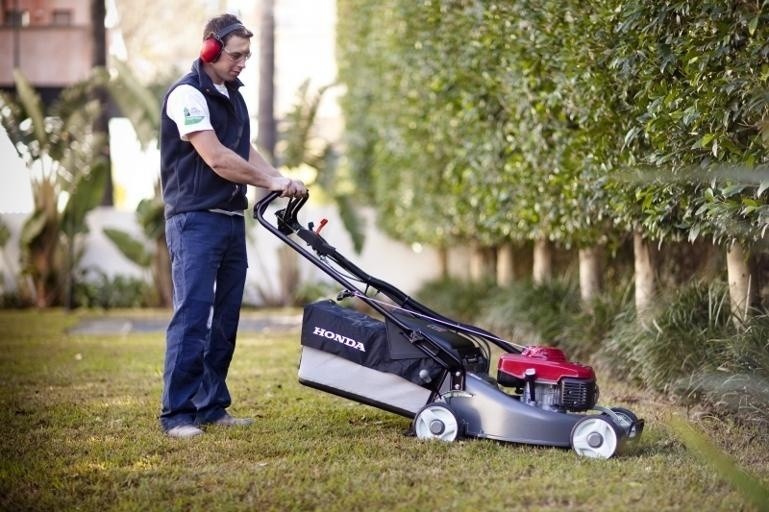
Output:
[222, 46, 252, 62]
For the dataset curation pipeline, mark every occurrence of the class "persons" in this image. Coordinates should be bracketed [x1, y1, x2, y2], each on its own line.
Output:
[153, 13, 308, 436]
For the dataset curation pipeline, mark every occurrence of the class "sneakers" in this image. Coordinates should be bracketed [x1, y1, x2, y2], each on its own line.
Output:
[168, 423, 204, 439]
[212, 412, 255, 427]
